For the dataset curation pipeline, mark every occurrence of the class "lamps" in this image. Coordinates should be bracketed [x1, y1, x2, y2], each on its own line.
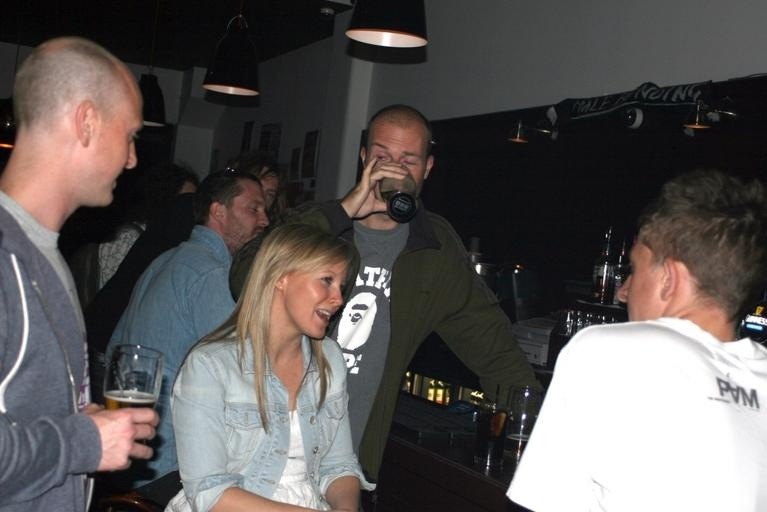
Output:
[344, 0, 427, 49]
[506, 118, 552, 144]
[682, 101, 739, 130]
[132, 64, 165, 129]
[201, 12, 262, 99]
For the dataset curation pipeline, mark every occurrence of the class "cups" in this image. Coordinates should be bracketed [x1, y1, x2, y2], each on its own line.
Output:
[378, 164, 417, 223]
[102, 344, 167, 477]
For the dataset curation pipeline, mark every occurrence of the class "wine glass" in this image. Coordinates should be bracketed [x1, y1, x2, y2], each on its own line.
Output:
[506, 384, 542, 471]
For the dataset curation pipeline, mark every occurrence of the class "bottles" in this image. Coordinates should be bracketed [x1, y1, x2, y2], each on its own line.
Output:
[560, 309, 618, 336]
[590, 224, 630, 307]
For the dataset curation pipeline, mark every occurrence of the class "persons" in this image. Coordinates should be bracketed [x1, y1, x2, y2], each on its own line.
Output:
[239, 157, 285, 212]
[0, 37, 160, 512]
[160, 224, 379, 512]
[236, 104, 551, 512]
[103, 171, 270, 508]
[502, 172, 763, 512]
[58, 160, 200, 405]
